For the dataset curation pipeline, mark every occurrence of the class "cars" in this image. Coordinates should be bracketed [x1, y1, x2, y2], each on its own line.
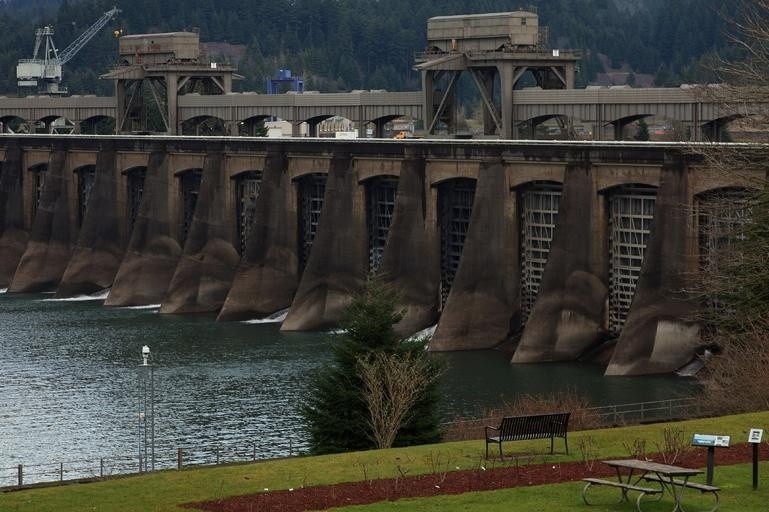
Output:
[395, 131, 407, 138]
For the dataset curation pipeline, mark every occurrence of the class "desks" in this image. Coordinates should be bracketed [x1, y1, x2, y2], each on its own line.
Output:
[600, 456, 706, 512]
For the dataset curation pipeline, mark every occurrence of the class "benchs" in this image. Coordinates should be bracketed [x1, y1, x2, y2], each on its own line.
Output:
[481, 410, 571, 465]
[637, 473, 722, 511]
[580, 477, 664, 511]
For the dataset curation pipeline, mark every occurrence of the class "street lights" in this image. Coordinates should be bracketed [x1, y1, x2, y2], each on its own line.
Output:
[137, 345, 156, 473]
